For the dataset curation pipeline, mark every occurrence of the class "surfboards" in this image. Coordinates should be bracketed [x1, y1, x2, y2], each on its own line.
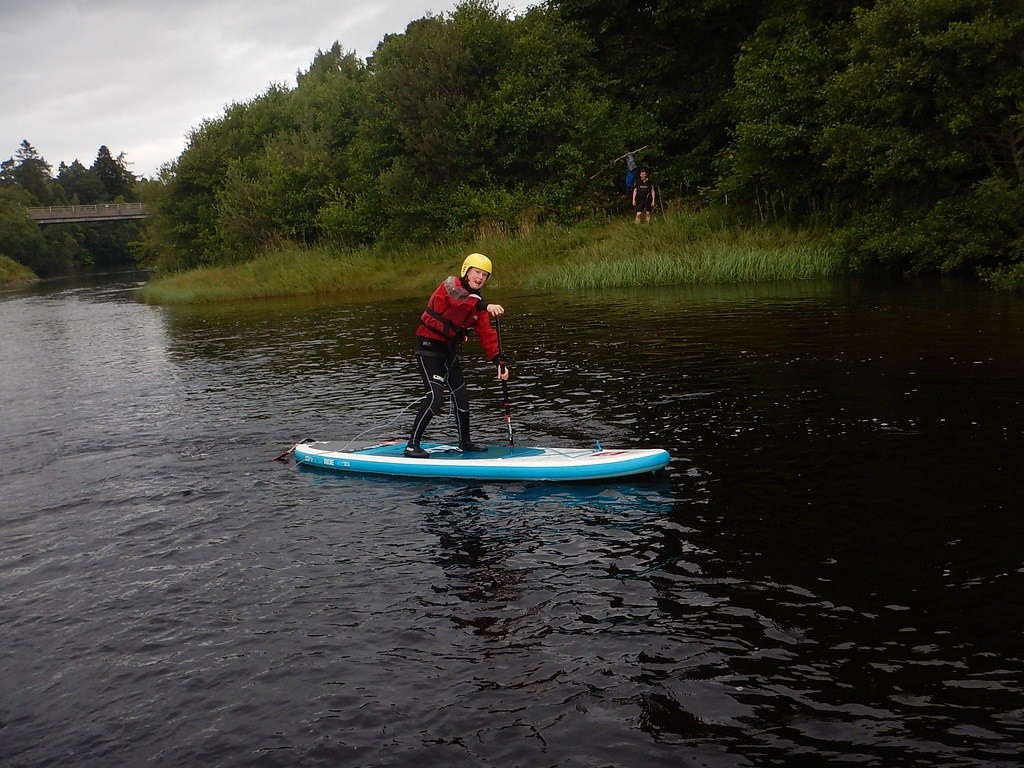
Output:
[294, 437, 671, 483]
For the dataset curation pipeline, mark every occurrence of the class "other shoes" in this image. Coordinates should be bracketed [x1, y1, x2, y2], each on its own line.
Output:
[405, 445, 430, 457]
[458, 443, 488, 451]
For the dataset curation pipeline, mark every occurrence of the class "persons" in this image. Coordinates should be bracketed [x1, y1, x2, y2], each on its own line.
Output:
[400, 253, 509, 459]
[632, 170, 656, 225]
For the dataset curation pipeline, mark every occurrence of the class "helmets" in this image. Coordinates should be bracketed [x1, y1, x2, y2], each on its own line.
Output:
[461, 252, 493, 285]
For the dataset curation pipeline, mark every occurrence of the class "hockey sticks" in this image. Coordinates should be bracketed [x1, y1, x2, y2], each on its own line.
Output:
[493, 309, 514, 446]
